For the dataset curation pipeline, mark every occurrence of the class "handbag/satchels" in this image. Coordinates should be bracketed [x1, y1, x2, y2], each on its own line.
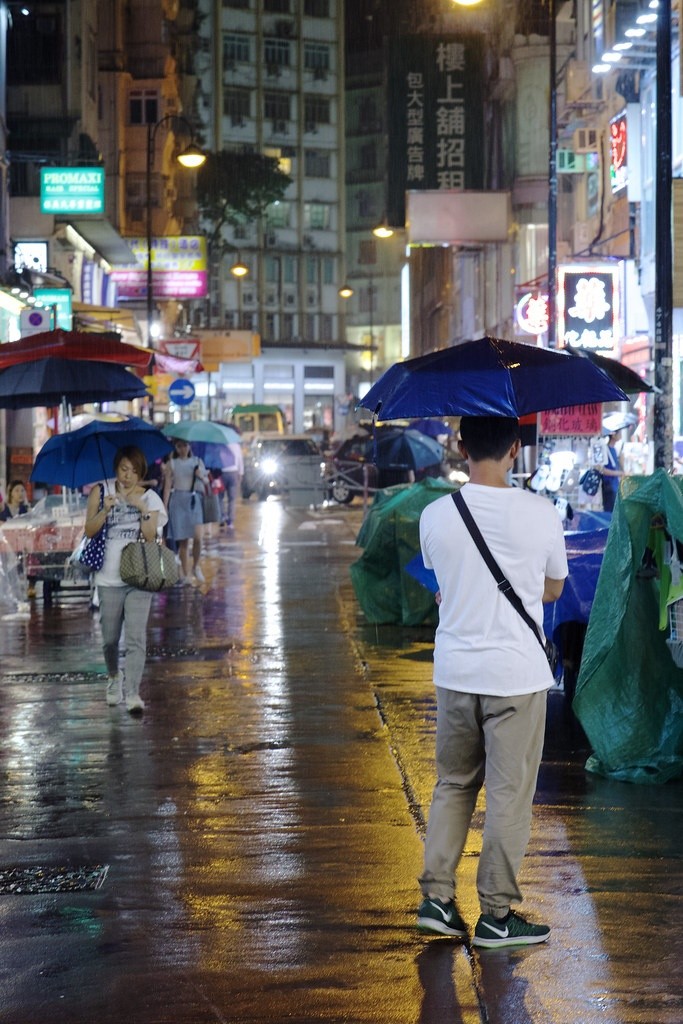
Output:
[578, 470, 602, 496]
[202, 483, 222, 523]
[120, 524, 178, 591]
[547, 638, 565, 686]
[69, 484, 108, 570]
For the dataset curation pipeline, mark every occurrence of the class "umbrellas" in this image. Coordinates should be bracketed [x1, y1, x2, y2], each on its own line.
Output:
[0, 358, 149, 432]
[29, 416, 175, 521]
[160, 419, 242, 443]
[0, 328, 157, 377]
[359, 334, 661, 422]
[192, 442, 235, 468]
[373, 428, 444, 471]
[408, 419, 455, 436]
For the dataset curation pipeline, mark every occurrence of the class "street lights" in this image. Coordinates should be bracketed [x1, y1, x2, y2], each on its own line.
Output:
[338, 269, 373, 388]
[146, 113, 205, 422]
[206, 241, 249, 421]
[453, 0, 560, 351]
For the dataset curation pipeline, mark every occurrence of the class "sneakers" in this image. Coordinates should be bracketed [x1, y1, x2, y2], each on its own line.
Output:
[125, 693, 145, 712]
[106, 669, 123, 705]
[472, 910, 549, 947]
[416, 896, 467, 937]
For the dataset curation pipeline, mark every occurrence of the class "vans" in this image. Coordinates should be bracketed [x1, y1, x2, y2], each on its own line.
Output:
[233, 405, 291, 459]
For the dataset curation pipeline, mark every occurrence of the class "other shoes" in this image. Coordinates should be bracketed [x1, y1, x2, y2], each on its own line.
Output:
[28, 589, 36, 596]
[183, 576, 194, 584]
[193, 566, 205, 582]
[219, 522, 225, 529]
[227, 523, 234, 529]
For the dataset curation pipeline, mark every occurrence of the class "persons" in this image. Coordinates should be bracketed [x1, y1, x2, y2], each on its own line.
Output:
[419, 416, 569, 947]
[203, 469, 223, 536]
[205, 442, 244, 528]
[84, 447, 168, 711]
[0, 479, 36, 596]
[219, 443, 243, 530]
[164, 439, 212, 584]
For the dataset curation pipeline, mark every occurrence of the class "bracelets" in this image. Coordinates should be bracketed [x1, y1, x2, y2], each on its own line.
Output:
[141, 513, 150, 520]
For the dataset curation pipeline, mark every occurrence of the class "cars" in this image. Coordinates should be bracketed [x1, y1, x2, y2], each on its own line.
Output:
[327, 436, 471, 505]
[240, 435, 327, 502]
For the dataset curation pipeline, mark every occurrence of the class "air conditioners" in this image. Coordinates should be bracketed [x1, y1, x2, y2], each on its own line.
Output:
[573, 128, 600, 153]
[556, 149, 584, 173]
[235, 228, 316, 307]
[225, 59, 325, 158]
